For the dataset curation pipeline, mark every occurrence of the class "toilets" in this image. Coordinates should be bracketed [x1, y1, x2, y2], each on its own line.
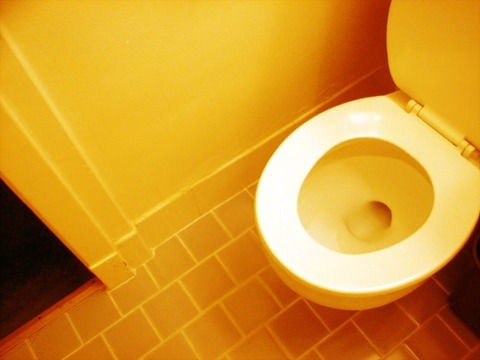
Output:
[254, 1, 479, 310]
